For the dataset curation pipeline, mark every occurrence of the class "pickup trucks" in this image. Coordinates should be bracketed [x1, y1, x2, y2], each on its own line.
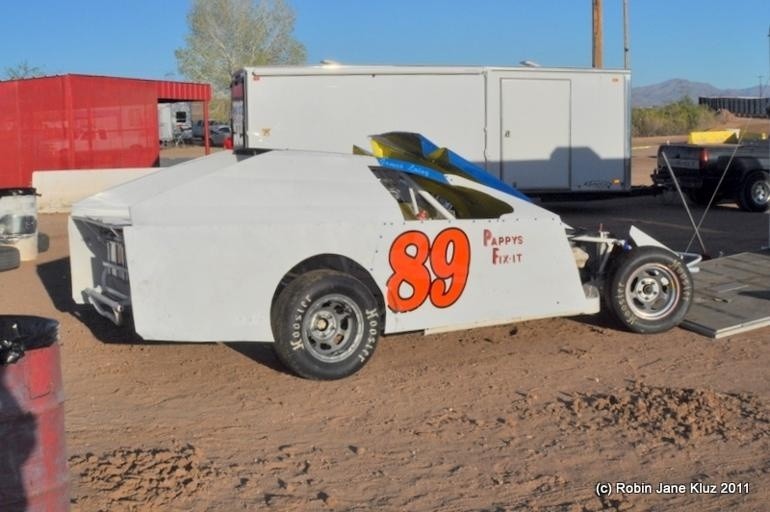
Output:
[648, 137, 770, 213]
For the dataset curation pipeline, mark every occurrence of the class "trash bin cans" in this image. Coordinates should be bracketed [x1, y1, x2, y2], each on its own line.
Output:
[0, 314, 70, 512]
[0, 188, 39, 262]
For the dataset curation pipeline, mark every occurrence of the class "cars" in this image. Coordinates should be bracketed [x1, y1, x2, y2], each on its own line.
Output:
[67, 130, 695, 378]
[193, 119, 231, 146]
